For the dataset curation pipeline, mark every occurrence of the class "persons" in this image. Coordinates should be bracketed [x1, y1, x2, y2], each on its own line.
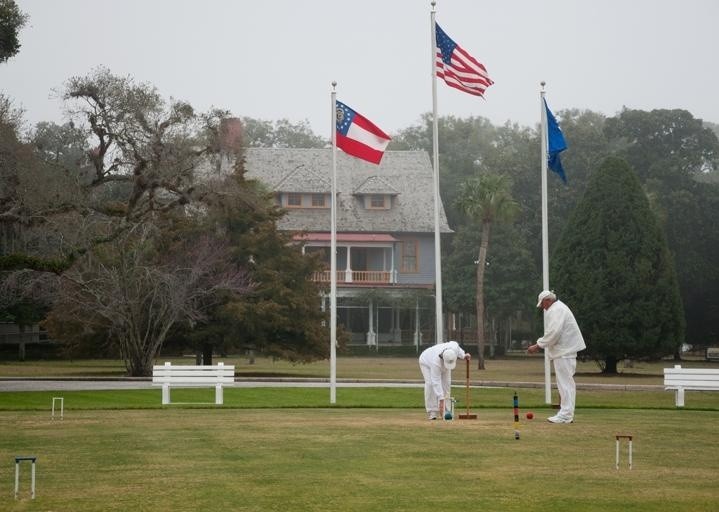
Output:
[528, 290, 587, 423]
[419, 341, 471, 420]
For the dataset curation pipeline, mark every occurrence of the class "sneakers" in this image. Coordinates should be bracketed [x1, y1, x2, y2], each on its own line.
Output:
[548, 416, 574, 424]
[428, 414, 436, 420]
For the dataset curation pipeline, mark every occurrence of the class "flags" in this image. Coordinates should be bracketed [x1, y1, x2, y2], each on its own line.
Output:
[336, 100, 392, 165]
[543, 98, 568, 183]
[436, 21, 494, 101]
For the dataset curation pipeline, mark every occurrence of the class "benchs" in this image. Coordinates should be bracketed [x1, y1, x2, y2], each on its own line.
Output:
[662, 362, 718, 407]
[151, 360, 237, 404]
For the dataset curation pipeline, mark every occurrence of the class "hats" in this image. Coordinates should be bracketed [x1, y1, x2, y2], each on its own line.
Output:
[536, 291, 553, 308]
[443, 350, 457, 370]
